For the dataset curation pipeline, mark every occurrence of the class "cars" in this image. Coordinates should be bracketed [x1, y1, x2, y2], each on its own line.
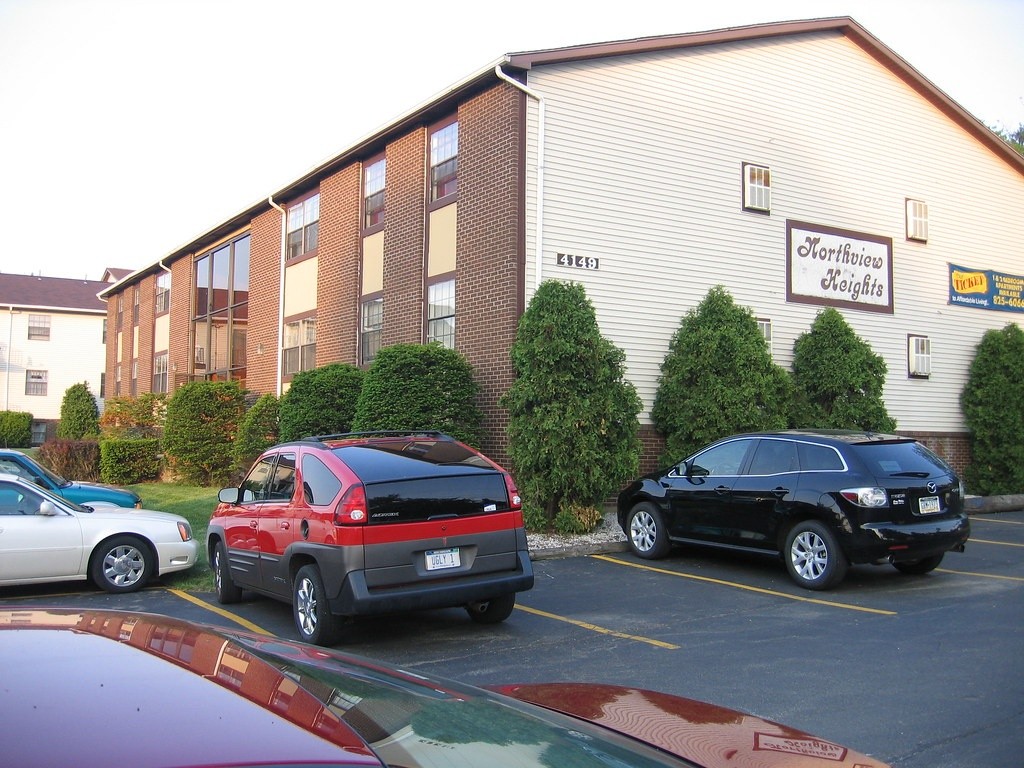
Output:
[0, 449, 144, 532]
[617, 429, 971, 592]
[0, 604, 893, 768]
[0, 473, 200, 595]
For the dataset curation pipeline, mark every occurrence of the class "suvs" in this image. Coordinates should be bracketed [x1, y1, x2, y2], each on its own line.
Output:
[204, 427, 535, 649]
